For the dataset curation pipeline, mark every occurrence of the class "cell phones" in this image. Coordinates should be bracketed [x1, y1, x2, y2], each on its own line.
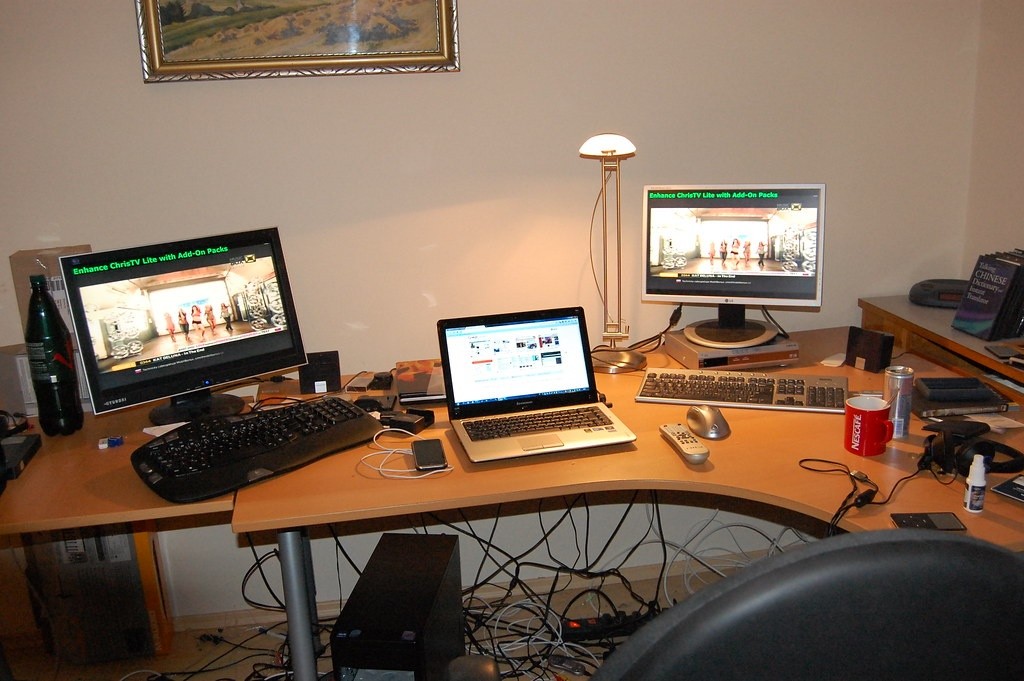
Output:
[411, 438, 448, 471]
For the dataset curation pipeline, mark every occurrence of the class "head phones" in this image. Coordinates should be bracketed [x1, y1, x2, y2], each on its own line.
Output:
[918, 429, 1024, 477]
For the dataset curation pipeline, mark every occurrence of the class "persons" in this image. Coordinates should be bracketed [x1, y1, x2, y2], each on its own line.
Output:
[177, 309, 192, 341]
[162, 313, 176, 342]
[718, 238, 729, 268]
[708, 241, 715, 265]
[743, 240, 751, 267]
[732, 238, 741, 270]
[756, 242, 766, 266]
[220, 303, 234, 330]
[191, 305, 206, 342]
[203, 305, 217, 335]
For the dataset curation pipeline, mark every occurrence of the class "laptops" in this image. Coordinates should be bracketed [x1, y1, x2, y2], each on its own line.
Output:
[437, 306, 637, 463]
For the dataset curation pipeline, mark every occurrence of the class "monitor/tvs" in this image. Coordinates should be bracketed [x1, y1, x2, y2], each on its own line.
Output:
[642, 184, 826, 349]
[58, 227, 309, 427]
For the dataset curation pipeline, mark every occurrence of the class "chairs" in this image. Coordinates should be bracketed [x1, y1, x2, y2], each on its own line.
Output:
[583, 530, 1024, 681]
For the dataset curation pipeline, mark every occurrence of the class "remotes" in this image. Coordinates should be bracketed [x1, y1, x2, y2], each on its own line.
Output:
[547, 656, 585, 675]
[659, 423, 710, 465]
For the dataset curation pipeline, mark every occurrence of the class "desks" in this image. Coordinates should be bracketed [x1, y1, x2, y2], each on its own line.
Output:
[0, 290, 1024, 680]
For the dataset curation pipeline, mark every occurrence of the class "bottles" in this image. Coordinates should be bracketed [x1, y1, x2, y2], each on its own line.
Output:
[963, 455, 987, 513]
[25, 272, 84, 437]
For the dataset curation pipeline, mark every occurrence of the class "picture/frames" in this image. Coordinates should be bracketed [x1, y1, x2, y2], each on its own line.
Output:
[133, 0, 463, 85]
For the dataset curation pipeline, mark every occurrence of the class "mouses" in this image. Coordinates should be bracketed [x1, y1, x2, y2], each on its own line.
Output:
[686, 405, 730, 438]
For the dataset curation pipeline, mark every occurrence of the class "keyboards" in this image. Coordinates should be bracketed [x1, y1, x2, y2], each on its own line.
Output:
[131, 394, 383, 503]
[634, 367, 849, 414]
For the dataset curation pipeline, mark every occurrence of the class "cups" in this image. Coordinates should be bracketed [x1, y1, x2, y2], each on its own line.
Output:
[843, 396, 895, 457]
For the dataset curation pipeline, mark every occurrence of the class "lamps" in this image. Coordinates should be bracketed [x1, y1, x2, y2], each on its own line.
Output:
[578, 133, 647, 374]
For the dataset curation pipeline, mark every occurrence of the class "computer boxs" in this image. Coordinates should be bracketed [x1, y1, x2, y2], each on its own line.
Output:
[663, 330, 800, 371]
[330, 533, 464, 681]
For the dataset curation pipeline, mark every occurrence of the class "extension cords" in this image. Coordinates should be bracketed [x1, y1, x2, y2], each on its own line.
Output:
[559, 611, 658, 641]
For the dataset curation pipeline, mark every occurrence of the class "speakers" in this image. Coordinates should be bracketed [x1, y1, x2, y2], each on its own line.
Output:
[298, 350, 341, 394]
[846, 326, 896, 374]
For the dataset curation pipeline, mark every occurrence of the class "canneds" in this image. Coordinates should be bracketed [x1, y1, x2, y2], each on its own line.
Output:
[882, 365, 914, 442]
